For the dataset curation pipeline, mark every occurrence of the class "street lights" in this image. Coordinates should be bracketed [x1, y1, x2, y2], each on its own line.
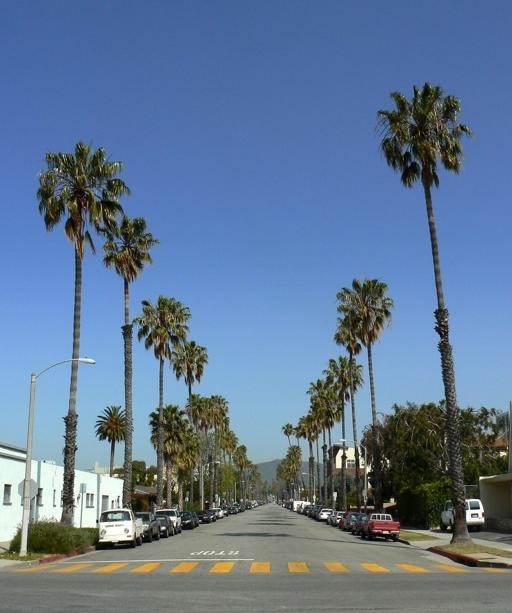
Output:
[235, 481, 245, 502]
[302, 473, 315, 505]
[340, 438, 367, 513]
[291, 483, 300, 501]
[18, 358, 96, 554]
[201, 461, 220, 509]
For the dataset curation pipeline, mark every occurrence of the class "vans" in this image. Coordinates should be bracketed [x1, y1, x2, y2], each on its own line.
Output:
[440, 499, 485, 533]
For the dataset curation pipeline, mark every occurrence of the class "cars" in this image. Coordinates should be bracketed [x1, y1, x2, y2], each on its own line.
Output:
[276, 499, 333, 520]
[197, 499, 263, 525]
[327, 510, 368, 535]
[96, 508, 200, 548]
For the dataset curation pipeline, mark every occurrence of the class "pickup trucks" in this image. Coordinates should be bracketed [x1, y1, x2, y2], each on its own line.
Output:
[362, 513, 401, 541]
